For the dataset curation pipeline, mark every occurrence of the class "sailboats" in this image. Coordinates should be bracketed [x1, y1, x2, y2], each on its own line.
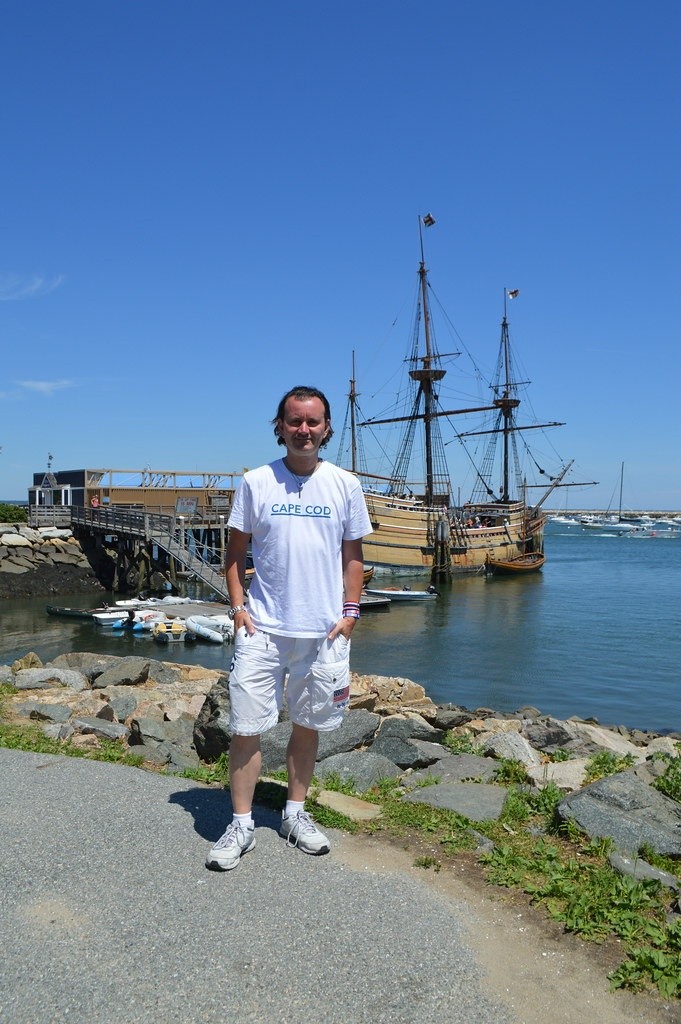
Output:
[547, 461, 681, 533]
[483, 477, 547, 577]
[335, 214, 574, 569]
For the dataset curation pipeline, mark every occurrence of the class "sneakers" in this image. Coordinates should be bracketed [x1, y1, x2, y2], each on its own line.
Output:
[279, 807, 331, 855]
[206, 820, 257, 872]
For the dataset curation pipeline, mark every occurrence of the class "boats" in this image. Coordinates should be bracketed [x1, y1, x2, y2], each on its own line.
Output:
[92, 595, 235, 643]
[365, 586, 438, 602]
[46, 603, 97, 619]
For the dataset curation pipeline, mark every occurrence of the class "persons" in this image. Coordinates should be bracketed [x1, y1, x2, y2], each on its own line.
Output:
[91, 494, 99, 520]
[205, 387, 374, 873]
[367, 490, 416, 510]
[450, 516, 492, 528]
[503, 518, 509, 526]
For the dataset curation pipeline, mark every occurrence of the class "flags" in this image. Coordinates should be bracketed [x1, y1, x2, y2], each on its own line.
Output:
[507, 288, 520, 300]
[423, 212, 435, 229]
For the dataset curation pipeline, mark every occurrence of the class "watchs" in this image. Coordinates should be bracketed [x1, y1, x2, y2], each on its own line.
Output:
[228, 605, 247, 621]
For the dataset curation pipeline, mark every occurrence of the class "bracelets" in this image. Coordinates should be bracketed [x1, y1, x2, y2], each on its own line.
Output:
[343, 600, 361, 619]
